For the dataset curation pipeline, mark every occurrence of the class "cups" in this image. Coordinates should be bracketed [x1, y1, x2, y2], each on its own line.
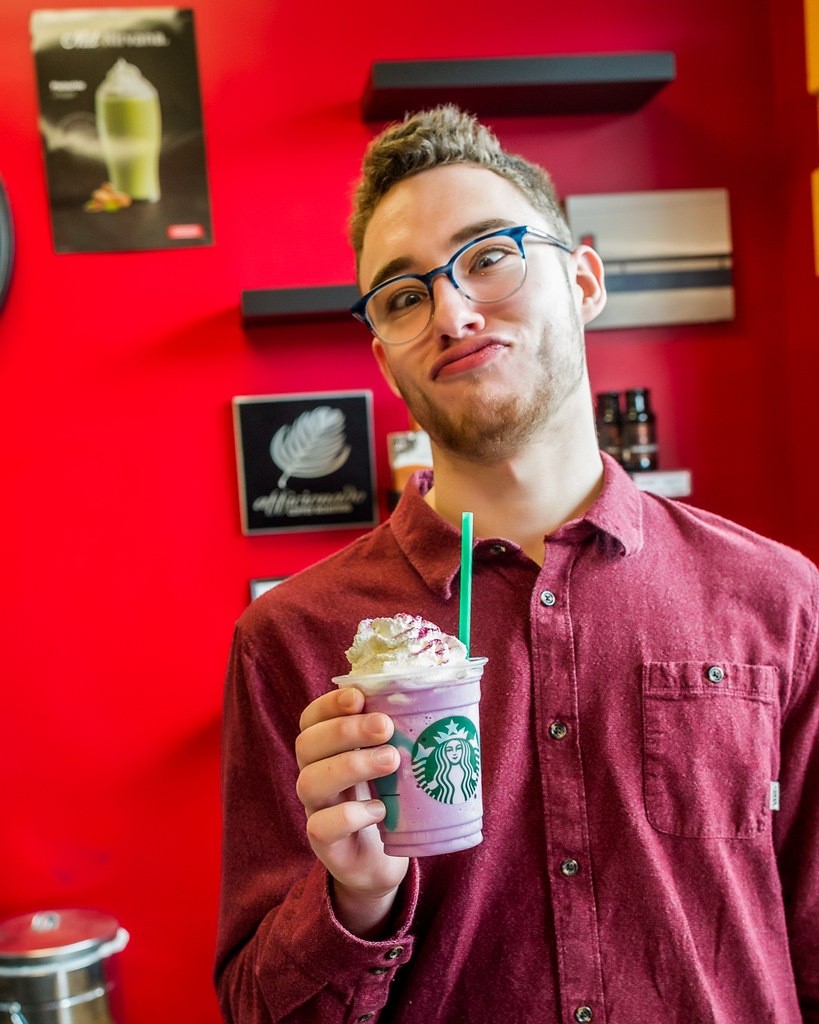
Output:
[94, 95, 163, 203]
[387, 432, 432, 494]
[329, 656, 491, 860]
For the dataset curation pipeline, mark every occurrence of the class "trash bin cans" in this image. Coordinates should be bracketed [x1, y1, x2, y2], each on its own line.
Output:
[0, 910, 134, 1024]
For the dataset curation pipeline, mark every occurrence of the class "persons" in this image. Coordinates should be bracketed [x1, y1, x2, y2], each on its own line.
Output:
[208, 107, 819, 1024]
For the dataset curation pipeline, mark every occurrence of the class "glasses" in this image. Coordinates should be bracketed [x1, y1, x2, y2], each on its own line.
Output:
[349, 225, 575, 345]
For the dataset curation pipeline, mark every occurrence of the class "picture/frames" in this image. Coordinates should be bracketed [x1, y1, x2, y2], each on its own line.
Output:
[230, 388, 380, 534]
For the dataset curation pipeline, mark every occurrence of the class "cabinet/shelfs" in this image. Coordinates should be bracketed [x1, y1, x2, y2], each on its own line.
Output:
[236, 51, 694, 501]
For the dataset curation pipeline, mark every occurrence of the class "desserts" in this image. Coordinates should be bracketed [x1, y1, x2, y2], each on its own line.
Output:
[342, 612, 469, 695]
[94, 58, 157, 100]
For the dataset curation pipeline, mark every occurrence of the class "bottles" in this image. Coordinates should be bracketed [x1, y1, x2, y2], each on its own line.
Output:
[622, 387, 658, 471]
[592, 391, 624, 467]
